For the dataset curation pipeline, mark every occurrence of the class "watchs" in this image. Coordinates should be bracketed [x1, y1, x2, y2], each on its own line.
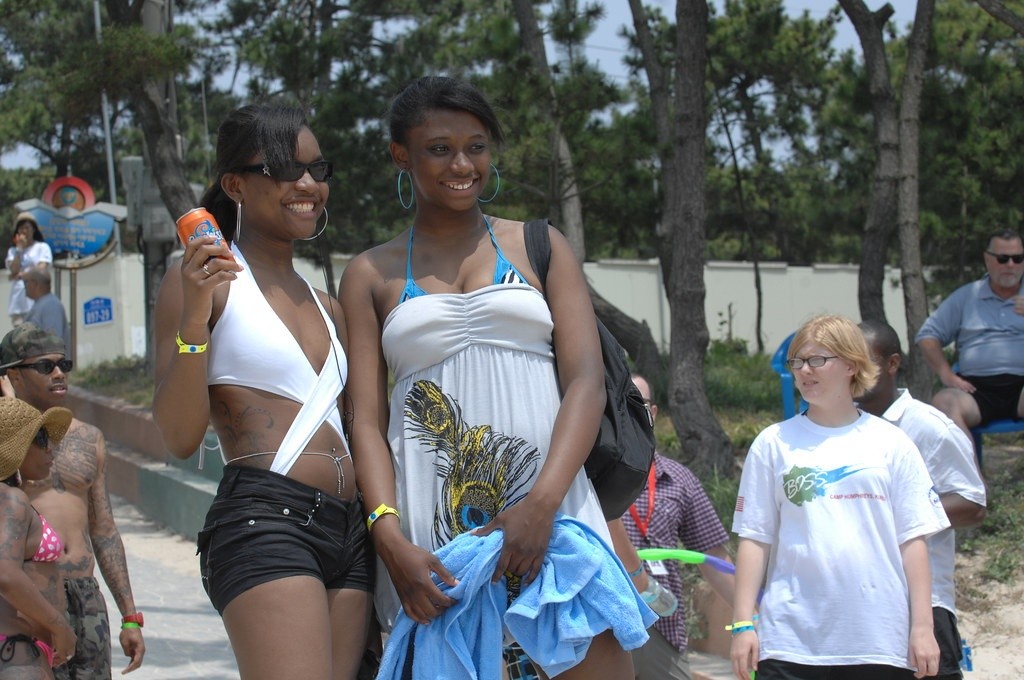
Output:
[121, 611, 144, 627]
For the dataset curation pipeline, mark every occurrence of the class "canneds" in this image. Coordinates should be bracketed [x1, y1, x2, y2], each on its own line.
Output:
[176, 207, 237, 272]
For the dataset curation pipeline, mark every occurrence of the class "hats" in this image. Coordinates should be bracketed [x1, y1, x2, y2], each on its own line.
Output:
[0, 396, 73, 483]
[0, 321, 65, 369]
[13, 211, 37, 231]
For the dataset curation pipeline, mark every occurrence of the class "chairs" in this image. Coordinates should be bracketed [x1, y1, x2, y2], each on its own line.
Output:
[771, 331, 810, 419]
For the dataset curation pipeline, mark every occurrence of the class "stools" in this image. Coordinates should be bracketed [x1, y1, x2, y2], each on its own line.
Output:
[952, 360, 1024, 469]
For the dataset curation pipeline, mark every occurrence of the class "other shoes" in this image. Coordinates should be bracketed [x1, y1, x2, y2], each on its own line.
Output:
[639, 576, 679, 618]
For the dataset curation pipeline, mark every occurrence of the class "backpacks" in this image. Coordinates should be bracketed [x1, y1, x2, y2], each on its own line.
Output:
[524, 217, 657, 522]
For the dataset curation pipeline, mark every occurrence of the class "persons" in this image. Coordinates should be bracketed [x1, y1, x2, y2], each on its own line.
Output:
[151, 100, 375, 680]
[729, 312, 952, 680]
[0, 321, 146, 680]
[606, 332, 758, 680]
[336, 75, 637, 680]
[4, 211, 53, 329]
[1, 397, 78, 680]
[851, 317, 988, 680]
[913, 226, 1024, 487]
[13, 267, 71, 374]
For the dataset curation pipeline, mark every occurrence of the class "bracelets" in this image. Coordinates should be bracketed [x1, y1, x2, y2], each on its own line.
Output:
[752, 613, 759, 621]
[120, 622, 140, 629]
[628, 560, 645, 578]
[175, 330, 208, 354]
[366, 503, 400, 534]
[725, 622, 753, 639]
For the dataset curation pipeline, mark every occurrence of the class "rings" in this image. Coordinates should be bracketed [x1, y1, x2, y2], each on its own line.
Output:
[202, 263, 212, 277]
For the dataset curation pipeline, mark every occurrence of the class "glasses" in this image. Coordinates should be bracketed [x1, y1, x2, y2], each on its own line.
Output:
[986, 250, 1024, 264]
[787, 356, 838, 369]
[245, 160, 333, 182]
[34, 426, 49, 448]
[15, 359, 74, 375]
